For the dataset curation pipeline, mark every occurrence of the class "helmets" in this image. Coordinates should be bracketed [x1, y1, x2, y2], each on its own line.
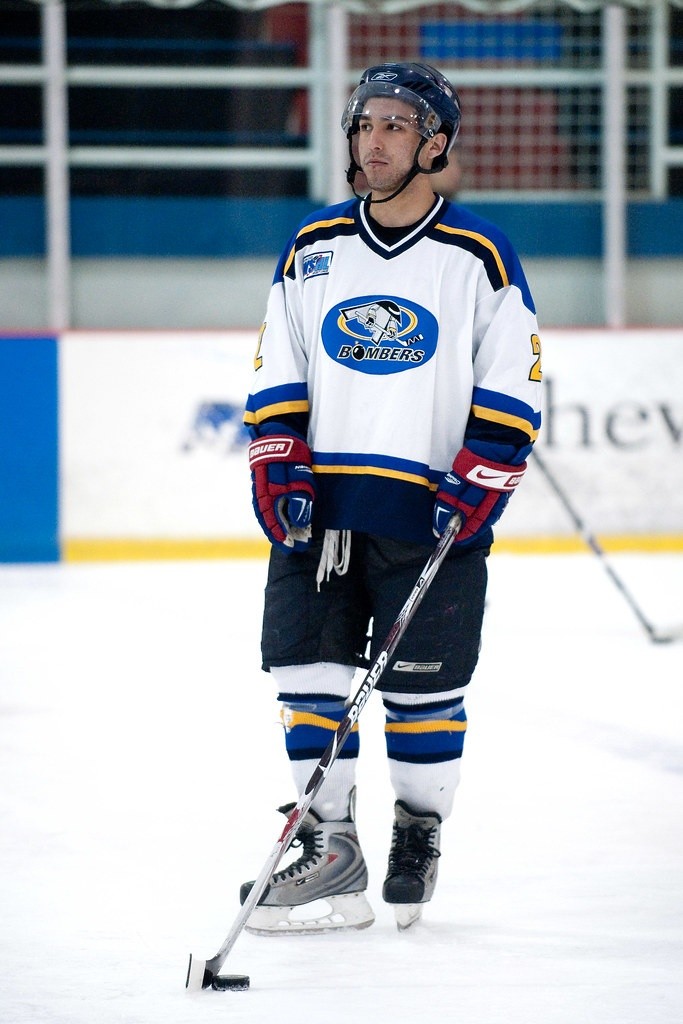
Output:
[358, 63, 461, 135]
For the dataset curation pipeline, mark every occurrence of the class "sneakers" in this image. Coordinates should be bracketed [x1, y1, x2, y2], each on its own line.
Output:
[382, 799, 441, 929]
[240, 785, 375, 932]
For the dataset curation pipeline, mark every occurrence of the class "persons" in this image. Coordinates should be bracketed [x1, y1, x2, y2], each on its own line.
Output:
[240, 61, 541, 937]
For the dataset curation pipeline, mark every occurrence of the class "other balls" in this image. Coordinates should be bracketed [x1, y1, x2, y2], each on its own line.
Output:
[211, 974, 251, 991]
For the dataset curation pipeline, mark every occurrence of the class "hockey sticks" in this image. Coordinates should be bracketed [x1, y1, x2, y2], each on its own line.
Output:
[184, 514, 464, 991]
[530, 444, 682, 644]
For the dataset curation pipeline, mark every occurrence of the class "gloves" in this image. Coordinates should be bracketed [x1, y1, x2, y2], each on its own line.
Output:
[434, 440, 533, 550]
[248, 422, 317, 555]
